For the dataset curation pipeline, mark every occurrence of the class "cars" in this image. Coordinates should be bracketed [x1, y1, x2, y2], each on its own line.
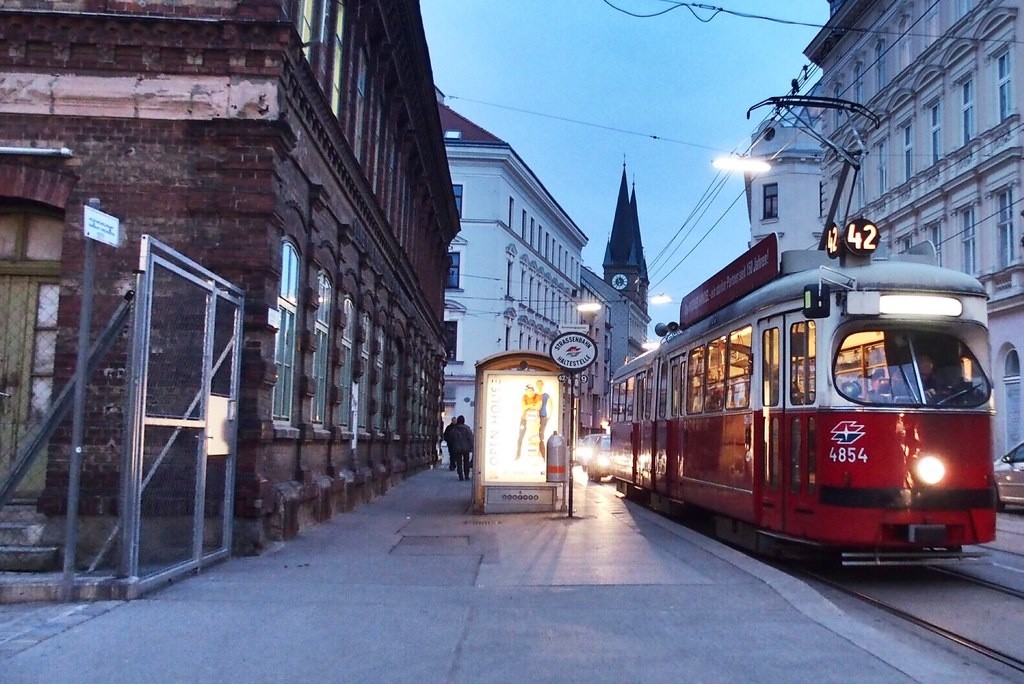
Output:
[579, 432, 611, 483]
[994, 442, 1024, 512]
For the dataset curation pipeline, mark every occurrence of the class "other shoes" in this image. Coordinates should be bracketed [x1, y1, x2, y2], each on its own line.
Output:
[466, 477, 470, 480]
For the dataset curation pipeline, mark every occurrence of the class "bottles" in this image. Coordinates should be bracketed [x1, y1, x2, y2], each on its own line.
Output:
[547, 431, 566, 481]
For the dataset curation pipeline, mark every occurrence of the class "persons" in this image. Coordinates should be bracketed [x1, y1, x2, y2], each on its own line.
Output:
[516, 380, 553, 462]
[858, 352, 988, 405]
[705, 388, 725, 409]
[772, 371, 803, 407]
[444, 415, 474, 481]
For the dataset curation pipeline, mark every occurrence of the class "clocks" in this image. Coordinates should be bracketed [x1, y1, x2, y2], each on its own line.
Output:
[612, 274, 627, 290]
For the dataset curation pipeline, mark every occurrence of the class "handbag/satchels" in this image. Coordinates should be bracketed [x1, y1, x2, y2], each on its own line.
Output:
[470, 452, 473, 469]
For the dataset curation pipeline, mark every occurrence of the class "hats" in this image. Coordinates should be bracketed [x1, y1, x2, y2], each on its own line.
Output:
[457, 415, 464, 423]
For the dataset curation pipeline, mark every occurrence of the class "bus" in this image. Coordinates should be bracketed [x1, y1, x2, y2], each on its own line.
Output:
[608, 91, 1001, 569]
